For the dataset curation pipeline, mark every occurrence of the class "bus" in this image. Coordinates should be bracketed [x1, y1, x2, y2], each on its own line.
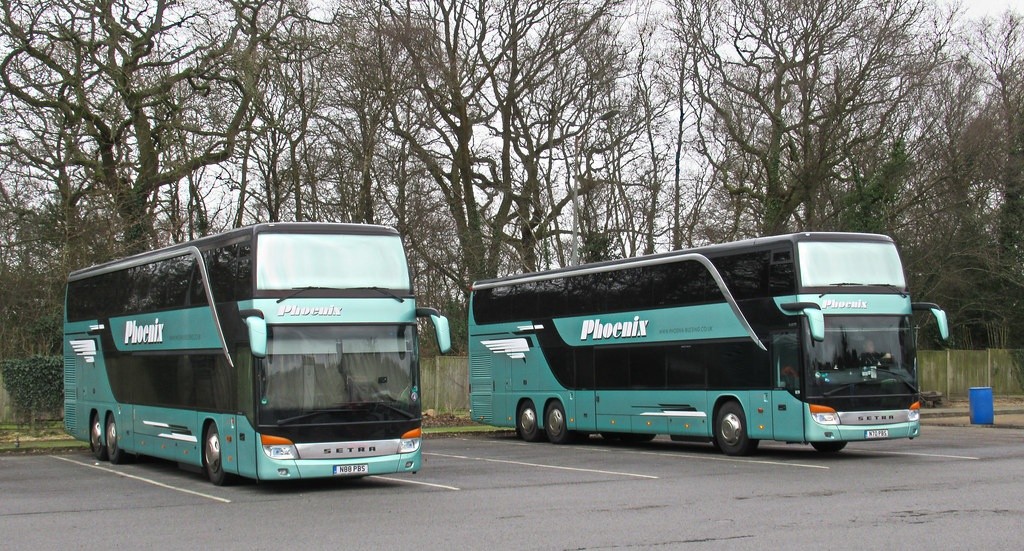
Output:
[63, 221, 452, 486]
[468, 232, 950, 457]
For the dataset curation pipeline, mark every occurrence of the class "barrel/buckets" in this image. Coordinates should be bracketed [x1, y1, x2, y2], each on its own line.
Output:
[968, 386, 994, 424]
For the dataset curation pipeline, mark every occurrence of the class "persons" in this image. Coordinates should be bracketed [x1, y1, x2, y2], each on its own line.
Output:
[858, 338, 875, 360]
